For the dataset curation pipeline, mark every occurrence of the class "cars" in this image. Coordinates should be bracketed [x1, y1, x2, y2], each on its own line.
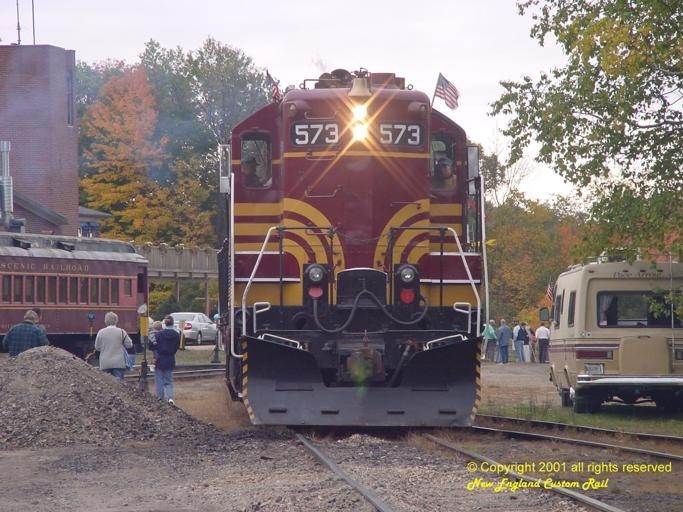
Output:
[160, 311, 217, 345]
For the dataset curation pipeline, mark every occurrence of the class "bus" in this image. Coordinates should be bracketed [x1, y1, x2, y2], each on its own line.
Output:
[536, 253, 683, 415]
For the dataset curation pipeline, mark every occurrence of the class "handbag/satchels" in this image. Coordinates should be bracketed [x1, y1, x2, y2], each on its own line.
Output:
[122, 345, 133, 369]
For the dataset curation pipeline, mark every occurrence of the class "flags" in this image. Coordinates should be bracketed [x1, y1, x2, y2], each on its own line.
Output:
[434, 72, 458, 110]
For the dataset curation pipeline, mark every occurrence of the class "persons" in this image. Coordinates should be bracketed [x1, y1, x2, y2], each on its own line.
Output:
[147, 315, 180, 405]
[94, 311, 133, 380]
[31, 308, 47, 336]
[148, 320, 163, 372]
[2, 310, 50, 359]
[242, 152, 259, 189]
[481, 318, 550, 363]
[432, 157, 457, 190]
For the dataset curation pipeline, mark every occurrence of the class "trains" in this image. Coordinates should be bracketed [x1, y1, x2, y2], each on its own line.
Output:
[214, 65, 490, 431]
[0, 229, 151, 365]
